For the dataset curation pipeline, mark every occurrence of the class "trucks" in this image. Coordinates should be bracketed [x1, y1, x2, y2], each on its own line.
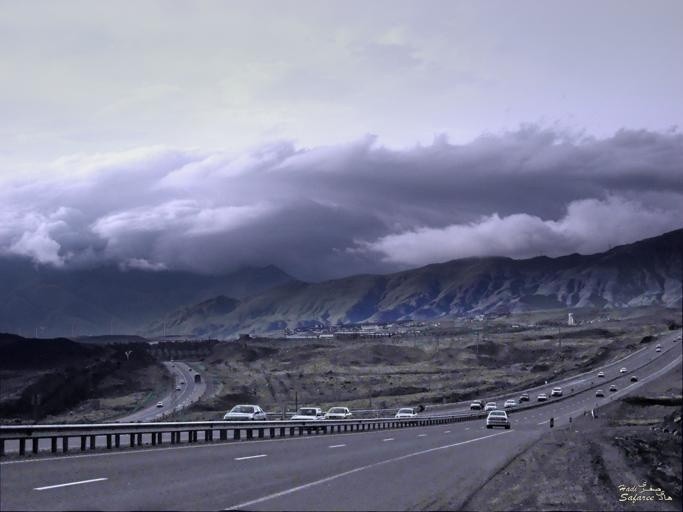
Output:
[193, 372, 201, 383]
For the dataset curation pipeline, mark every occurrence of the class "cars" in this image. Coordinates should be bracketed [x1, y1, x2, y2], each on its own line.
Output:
[394, 407, 418, 419]
[223, 404, 355, 429]
[595, 384, 617, 397]
[597, 372, 605, 378]
[175, 379, 185, 390]
[655, 344, 661, 352]
[630, 375, 638, 382]
[469, 386, 564, 430]
[156, 402, 163, 407]
[620, 367, 627, 373]
